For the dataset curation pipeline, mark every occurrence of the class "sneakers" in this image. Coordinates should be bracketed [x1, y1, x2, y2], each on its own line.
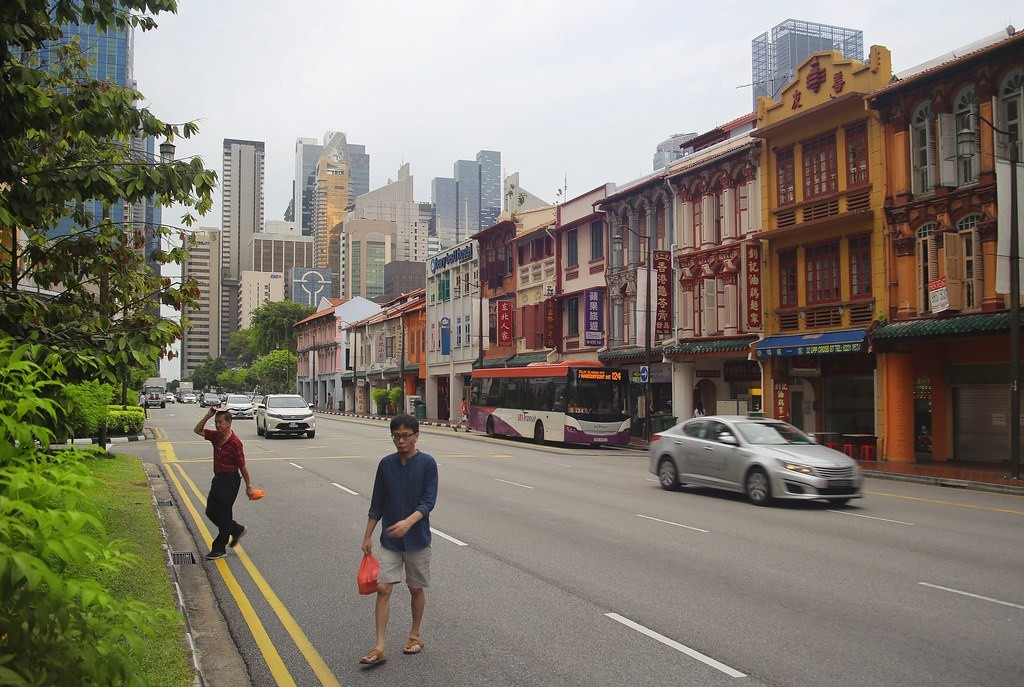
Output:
[229, 526, 247, 547]
[205, 548, 228, 560]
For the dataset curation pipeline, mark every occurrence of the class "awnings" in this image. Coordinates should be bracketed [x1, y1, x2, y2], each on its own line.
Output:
[755, 330, 869, 358]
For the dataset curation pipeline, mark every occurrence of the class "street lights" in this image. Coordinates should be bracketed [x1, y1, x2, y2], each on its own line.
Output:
[299, 332, 315, 404]
[276, 341, 290, 395]
[382, 303, 405, 414]
[338, 320, 356, 413]
[957, 112, 1021, 479]
[612, 224, 651, 440]
[454, 278, 483, 369]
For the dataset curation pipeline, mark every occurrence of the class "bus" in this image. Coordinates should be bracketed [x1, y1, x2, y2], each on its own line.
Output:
[468, 361, 632, 449]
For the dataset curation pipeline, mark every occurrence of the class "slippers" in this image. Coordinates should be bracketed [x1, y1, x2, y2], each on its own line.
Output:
[404, 637, 424, 653]
[359, 649, 387, 664]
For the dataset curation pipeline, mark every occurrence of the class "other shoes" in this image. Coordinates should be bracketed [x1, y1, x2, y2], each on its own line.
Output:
[466, 429, 471, 432]
[453, 426, 457, 432]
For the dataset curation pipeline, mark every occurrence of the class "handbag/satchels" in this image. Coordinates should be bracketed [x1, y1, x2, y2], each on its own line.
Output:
[357, 551, 380, 593]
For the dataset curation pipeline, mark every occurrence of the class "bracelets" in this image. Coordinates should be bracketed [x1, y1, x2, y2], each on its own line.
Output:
[248, 484, 252, 487]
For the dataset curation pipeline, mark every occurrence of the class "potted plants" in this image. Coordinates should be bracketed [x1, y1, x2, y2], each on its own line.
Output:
[388, 387, 404, 415]
[370, 388, 390, 414]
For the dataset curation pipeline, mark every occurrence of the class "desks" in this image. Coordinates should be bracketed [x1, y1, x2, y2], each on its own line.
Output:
[813, 431, 840, 446]
[842, 433, 874, 458]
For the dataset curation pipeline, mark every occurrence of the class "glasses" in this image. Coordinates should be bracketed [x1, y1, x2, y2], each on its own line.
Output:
[392, 430, 418, 442]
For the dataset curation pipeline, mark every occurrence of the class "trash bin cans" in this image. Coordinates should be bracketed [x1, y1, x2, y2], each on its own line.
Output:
[338, 401, 345, 411]
[414, 404, 426, 419]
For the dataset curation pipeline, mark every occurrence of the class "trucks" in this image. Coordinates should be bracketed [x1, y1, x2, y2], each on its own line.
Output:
[142, 377, 167, 409]
[176, 382, 200, 403]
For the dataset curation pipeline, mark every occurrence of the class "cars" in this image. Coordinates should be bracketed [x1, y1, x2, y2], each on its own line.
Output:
[222, 395, 255, 420]
[199, 393, 222, 409]
[244, 392, 265, 415]
[184, 394, 200, 404]
[649, 415, 865, 507]
[166, 393, 175, 402]
[256, 394, 316, 439]
[218, 393, 236, 402]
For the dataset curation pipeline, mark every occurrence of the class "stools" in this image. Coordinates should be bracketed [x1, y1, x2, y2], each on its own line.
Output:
[826, 442, 877, 461]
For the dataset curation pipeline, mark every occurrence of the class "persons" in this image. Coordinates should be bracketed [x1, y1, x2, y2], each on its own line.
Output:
[360, 414, 438, 663]
[452, 397, 470, 432]
[598, 400, 605, 409]
[314, 393, 318, 408]
[693, 401, 706, 418]
[328, 393, 333, 414]
[221, 392, 227, 401]
[194, 406, 254, 559]
[140, 392, 149, 420]
